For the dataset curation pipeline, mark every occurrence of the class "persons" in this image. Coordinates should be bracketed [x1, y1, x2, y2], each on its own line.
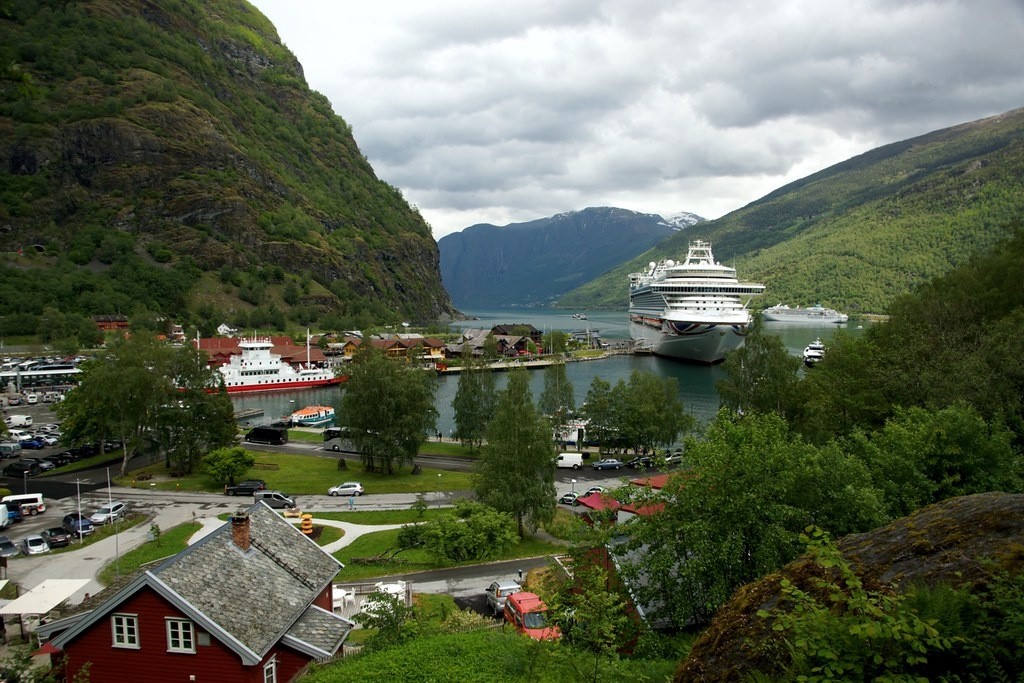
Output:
[28, 388, 36, 394]
[292, 348, 356, 372]
[435, 429, 439, 439]
[563, 441, 566, 450]
[452, 431, 459, 441]
[23, 389, 26, 395]
[3, 406, 7, 415]
[439, 432, 442, 443]
[348, 494, 356, 510]
[421, 351, 561, 369]
[518, 567, 523, 581]
[578, 441, 580, 451]
[643, 448, 647, 455]
[666, 448, 670, 454]
[564, 334, 636, 359]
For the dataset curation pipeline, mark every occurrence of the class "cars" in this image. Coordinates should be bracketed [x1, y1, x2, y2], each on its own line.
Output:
[0, 353, 128, 558]
[327, 481, 365, 496]
[590, 459, 623, 471]
[558, 492, 580, 507]
[661, 452, 683, 464]
[626, 455, 655, 468]
[586, 486, 605, 497]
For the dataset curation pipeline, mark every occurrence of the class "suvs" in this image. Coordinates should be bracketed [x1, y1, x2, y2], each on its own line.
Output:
[504, 591, 563, 642]
[225, 479, 296, 510]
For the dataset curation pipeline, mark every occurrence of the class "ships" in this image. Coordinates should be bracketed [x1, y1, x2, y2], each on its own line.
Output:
[170, 326, 350, 395]
[624, 239, 768, 363]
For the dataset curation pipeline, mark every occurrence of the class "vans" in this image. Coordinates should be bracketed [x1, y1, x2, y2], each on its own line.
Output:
[551, 453, 584, 470]
[244, 426, 288, 445]
[485, 580, 525, 617]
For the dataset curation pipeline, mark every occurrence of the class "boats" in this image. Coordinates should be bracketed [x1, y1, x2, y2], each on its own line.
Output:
[630, 314, 662, 329]
[802, 337, 829, 367]
[572, 313, 588, 321]
[761, 301, 848, 324]
[285, 405, 336, 426]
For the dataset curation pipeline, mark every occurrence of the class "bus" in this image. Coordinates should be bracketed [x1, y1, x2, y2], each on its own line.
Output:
[323, 427, 383, 455]
